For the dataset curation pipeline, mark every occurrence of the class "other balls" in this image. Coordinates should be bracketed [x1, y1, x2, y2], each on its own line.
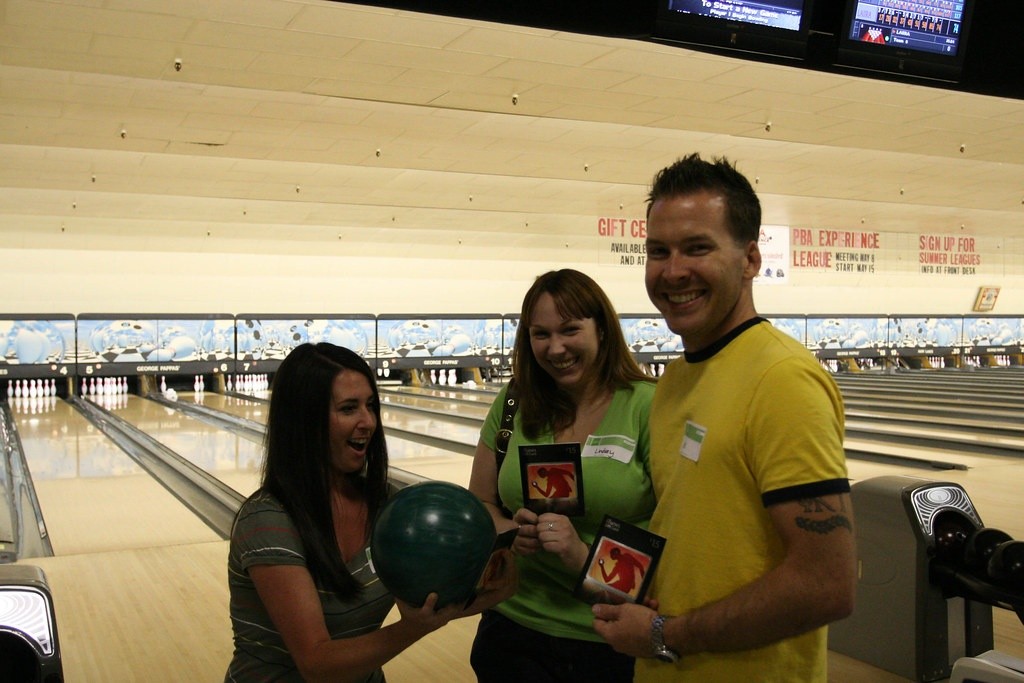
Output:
[980, 535, 1002, 559]
[1007, 554, 1024, 582]
[369, 480, 499, 612]
[934, 520, 969, 558]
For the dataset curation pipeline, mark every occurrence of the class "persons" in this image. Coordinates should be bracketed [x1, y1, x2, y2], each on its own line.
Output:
[468, 268, 660, 683]
[225, 342, 517, 683]
[532, 468, 575, 499]
[593, 153, 856, 683]
[598, 548, 644, 593]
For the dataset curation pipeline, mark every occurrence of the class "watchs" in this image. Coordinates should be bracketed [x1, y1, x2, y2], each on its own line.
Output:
[650, 615, 680, 663]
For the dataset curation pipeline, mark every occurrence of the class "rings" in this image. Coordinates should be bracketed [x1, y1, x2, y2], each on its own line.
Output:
[549, 521, 553, 530]
[518, 524, 522, 535]
